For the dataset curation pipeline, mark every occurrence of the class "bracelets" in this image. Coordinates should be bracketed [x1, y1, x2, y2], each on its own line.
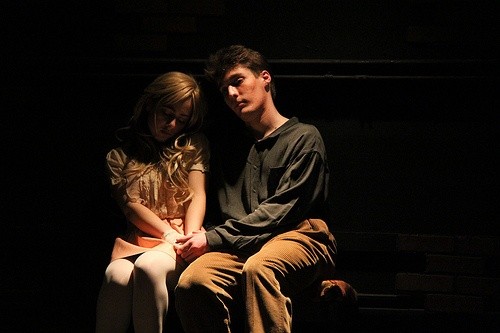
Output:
[161, 228, 175, 238]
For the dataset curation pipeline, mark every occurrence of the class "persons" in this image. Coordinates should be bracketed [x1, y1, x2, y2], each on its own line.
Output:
[94, 70, 214, 332]
[176, 44, 338, 333]
